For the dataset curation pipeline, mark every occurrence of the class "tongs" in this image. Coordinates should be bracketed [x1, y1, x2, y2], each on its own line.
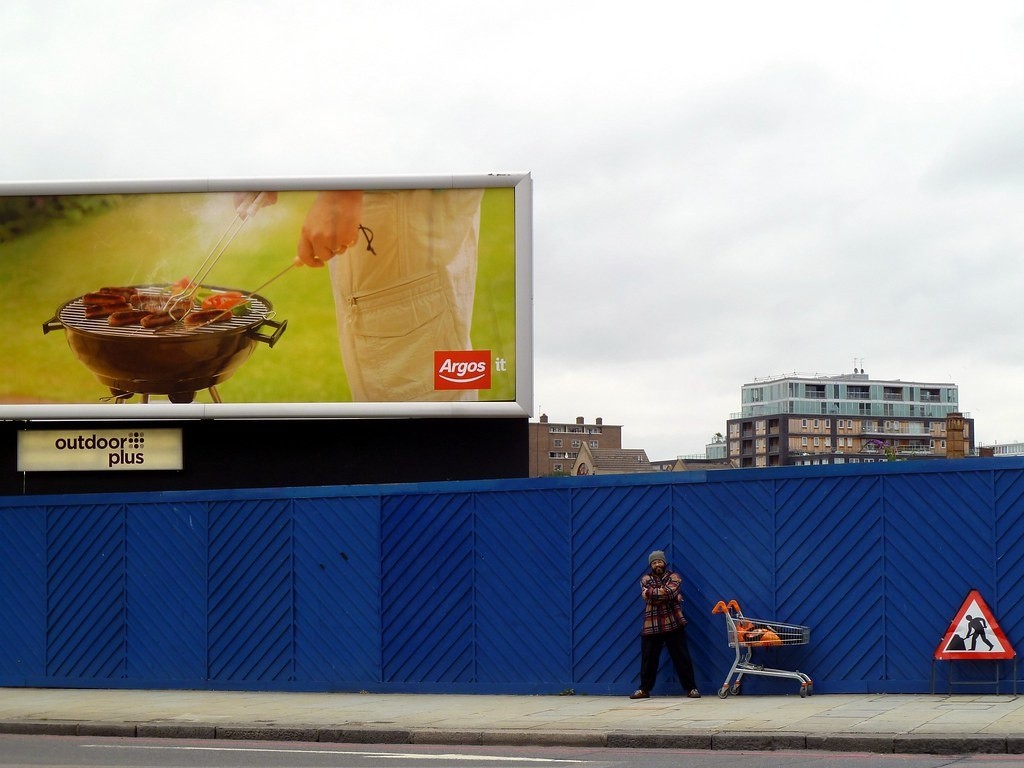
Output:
[162, 192, 269, 322]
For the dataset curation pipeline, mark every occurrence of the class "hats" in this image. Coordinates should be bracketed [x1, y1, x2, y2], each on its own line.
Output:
[649, 550, 667, 565]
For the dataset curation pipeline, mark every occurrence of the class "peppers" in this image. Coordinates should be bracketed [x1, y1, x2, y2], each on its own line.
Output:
[195, 288, 245, 311]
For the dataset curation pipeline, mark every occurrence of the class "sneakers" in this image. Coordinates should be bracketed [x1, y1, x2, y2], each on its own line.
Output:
[629, 690, 649, 699]
[688, 689, 701, 698]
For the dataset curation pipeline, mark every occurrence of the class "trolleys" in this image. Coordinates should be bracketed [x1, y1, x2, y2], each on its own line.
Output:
[712, 599, 814, 697]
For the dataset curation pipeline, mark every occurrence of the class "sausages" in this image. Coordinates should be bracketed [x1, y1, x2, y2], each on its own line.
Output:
[82, 286, 232, 327]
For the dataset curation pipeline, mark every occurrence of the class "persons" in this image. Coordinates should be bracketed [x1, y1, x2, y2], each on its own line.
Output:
[629, 550, 701, 701]
[234, 186, 484, 402]
